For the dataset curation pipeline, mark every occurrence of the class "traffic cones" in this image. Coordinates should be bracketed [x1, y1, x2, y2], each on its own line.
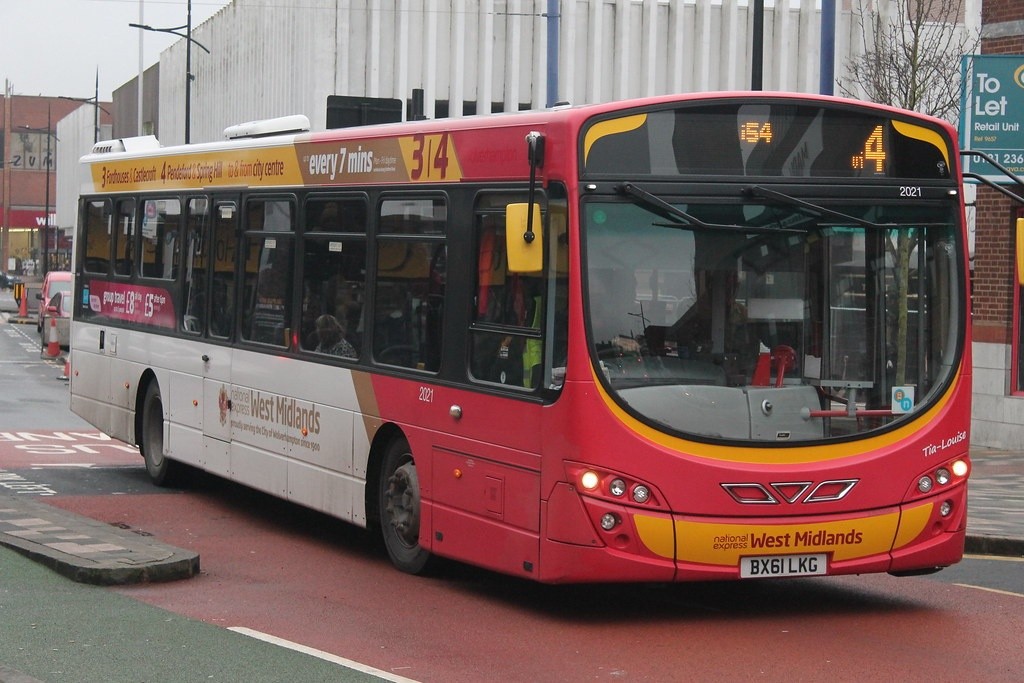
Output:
[14, 285, 32, 319]
[57, 349, 71, 380]
[40, 316, 65, 360]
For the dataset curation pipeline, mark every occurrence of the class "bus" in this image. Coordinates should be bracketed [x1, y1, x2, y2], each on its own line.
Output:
[67, 89, 1024, 594]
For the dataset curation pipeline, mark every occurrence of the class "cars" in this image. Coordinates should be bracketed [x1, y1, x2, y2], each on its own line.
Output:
[40, 291, 70, 342]
[0, 271, 23, 289]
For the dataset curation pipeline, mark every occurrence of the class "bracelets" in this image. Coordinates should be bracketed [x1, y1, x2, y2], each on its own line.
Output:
[613, 336, 619, 347]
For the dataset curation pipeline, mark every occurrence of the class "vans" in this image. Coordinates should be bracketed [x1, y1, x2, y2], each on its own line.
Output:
[34, 271, 72, 334]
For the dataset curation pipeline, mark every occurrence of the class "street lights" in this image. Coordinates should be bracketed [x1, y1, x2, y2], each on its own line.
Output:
[55, 96, 111, 149]
[127, 21, 212, 149]
[18, 125, 60, 280]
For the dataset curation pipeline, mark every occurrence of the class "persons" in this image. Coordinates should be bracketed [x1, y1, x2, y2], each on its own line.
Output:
[588, 270, 641, 353]
[314, 314, 357, 359]
